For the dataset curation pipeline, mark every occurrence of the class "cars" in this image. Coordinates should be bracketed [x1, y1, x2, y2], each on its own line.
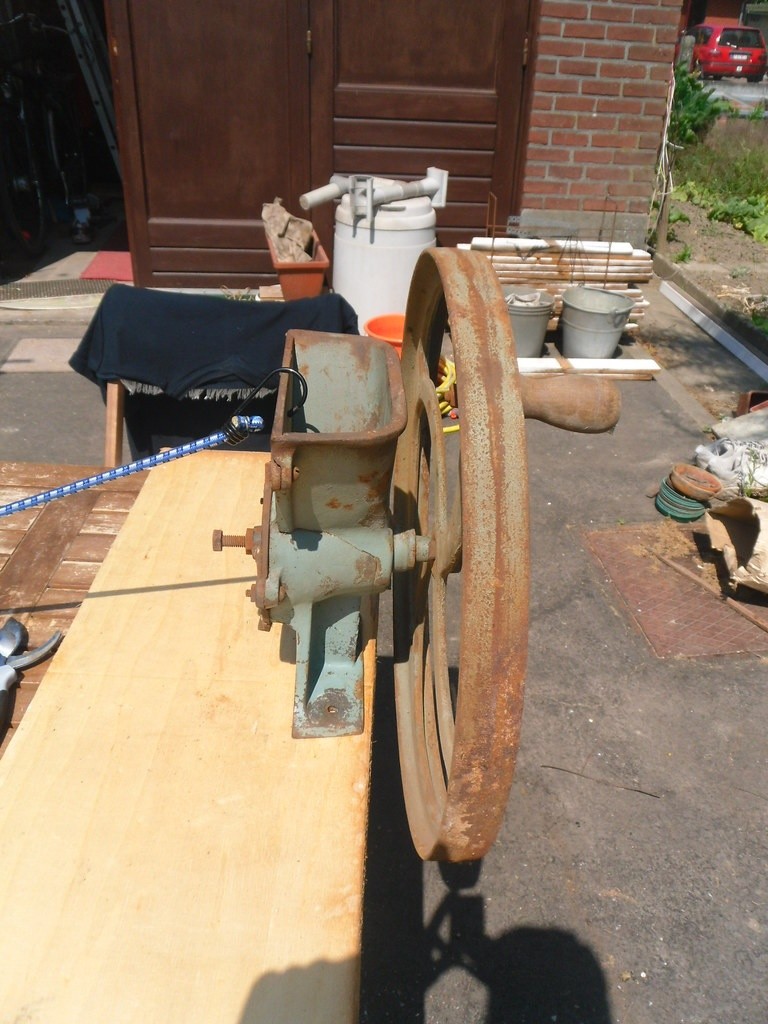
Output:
[673, 24, 768, 83]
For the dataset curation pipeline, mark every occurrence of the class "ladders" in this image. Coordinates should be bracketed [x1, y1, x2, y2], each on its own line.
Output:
[58, 0, 123, 179]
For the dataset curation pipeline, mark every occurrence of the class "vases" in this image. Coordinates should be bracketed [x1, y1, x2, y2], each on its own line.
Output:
[364, 314, 408, 360]
[263, 227, 330, 301]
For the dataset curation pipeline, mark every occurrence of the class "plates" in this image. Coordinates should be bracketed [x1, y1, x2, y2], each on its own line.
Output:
[655, 477, 706, 523]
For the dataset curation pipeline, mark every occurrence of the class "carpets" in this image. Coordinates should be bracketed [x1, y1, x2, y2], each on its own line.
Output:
[81, 249, 131, 280]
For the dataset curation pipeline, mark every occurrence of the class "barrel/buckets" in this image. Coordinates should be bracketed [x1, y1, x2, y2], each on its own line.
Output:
[502, 286, 555, 357]
[562, 282, 635, 358]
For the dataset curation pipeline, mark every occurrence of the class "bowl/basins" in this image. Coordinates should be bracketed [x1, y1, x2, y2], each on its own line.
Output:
[365, 315, 405, 360]
[671, 464, 723, 501]
